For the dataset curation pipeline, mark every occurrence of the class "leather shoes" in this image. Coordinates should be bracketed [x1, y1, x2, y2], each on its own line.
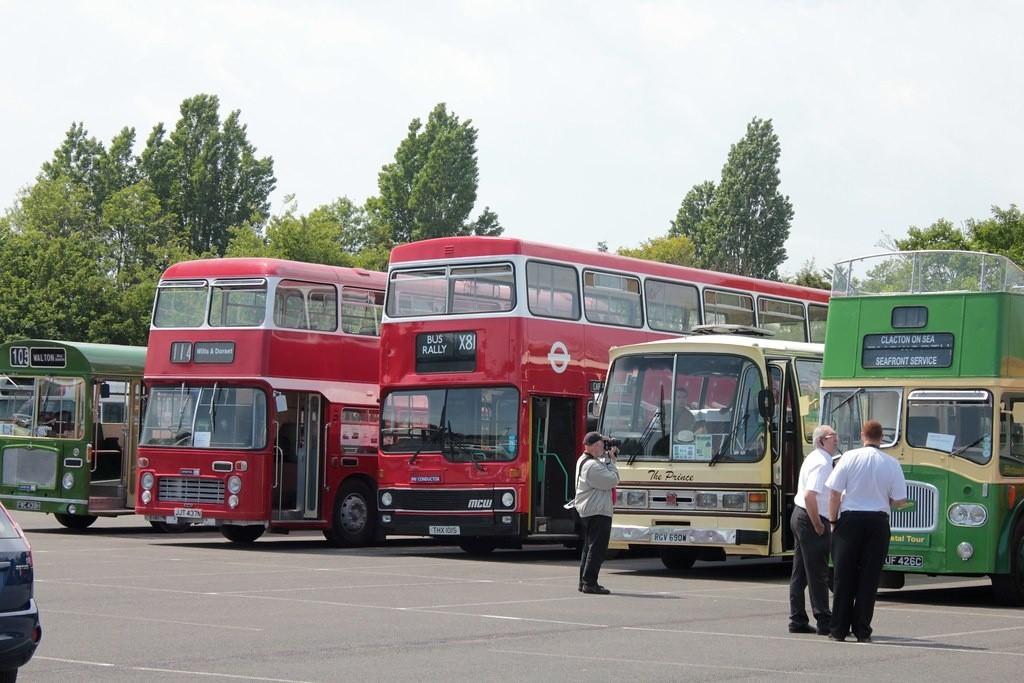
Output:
[855, 636, 872, 643]
[578, 582, 610, 595]
[827, 632, 846, 641]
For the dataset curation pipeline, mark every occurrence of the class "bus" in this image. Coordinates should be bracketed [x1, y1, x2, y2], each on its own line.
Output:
[819, 249, 1024, 599]
[0, 339, 158, 530]
[590, 321, 877, 572]
[378, 238, 860, 554]
[134, 259, 634, 541]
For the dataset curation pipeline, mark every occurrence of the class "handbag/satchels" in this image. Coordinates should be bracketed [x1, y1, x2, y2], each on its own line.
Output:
[563, 498, 581, 524]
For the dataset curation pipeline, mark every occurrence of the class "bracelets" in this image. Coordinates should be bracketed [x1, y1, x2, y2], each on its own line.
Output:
[829, 521, 837, 525]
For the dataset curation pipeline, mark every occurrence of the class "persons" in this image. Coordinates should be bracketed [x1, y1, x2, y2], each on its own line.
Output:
[788, 425, 838, 635]
[575, 431, 619, 595]
[824, 420, 907, 642]
[653, 388, 695, 436]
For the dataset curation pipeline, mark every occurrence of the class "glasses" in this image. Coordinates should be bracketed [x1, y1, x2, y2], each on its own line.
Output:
[825, 435, 840, 438]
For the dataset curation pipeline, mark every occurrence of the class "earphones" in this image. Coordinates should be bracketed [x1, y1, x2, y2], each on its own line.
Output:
[820, 441, 824, 446]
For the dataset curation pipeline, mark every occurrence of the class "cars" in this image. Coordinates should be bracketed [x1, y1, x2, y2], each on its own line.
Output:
[0, 503, 43, 683]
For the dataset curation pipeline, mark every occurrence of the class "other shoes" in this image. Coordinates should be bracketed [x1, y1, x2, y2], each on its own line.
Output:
[818, 626, 851, 636]
[790, 624, 816, 633]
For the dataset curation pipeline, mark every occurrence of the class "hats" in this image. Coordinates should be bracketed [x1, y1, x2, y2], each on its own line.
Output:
[583, 431, 609, 444]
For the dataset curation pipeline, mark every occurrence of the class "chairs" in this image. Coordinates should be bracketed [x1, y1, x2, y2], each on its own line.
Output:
[907, 416, 939, 448]
[705, 410, 730, 454]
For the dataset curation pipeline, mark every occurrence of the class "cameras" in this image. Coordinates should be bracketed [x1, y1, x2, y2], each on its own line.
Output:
[604, 440, 621, 451]
